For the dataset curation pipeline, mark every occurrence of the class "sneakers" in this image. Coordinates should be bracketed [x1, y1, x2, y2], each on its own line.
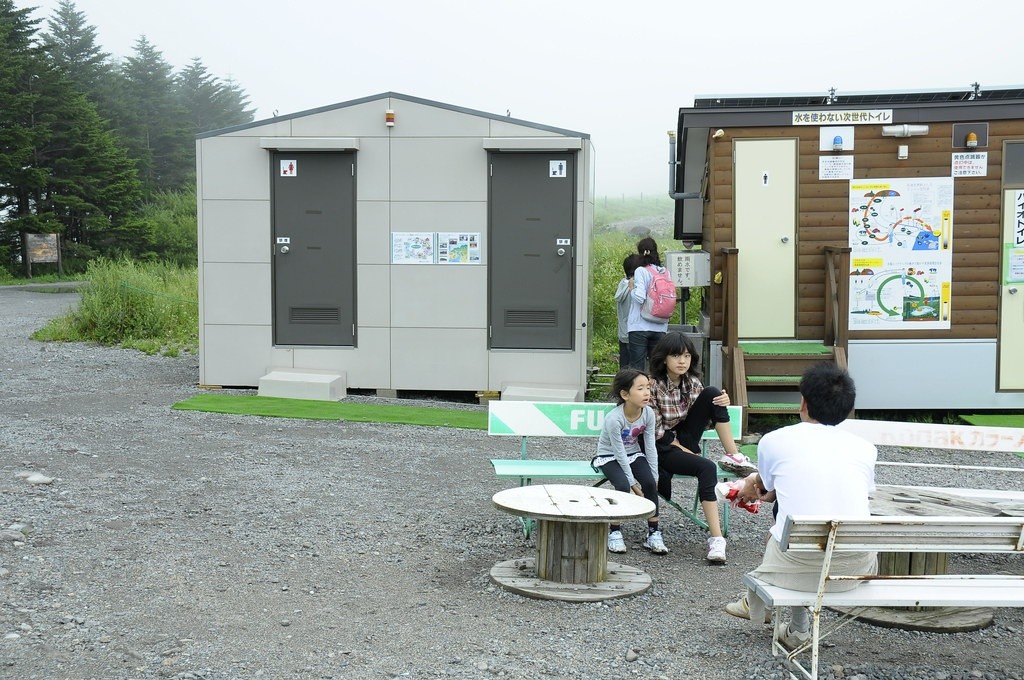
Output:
[643, 531, 668, 554]
[778, 622, 811, 651]
[718, 452, 759, 478]
[725, 588, 773, 623]
[707, 536, 727, 562]
[607, 531, 626, 552]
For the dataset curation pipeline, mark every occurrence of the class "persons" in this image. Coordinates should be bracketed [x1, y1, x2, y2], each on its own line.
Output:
[647, 331, 760, 562]
[726, 360, 879, 651]
[615, 237, 671, 374]
[593, 365, 669, 554]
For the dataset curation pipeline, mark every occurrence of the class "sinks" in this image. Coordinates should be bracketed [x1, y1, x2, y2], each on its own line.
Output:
[665, 324, 704, 365]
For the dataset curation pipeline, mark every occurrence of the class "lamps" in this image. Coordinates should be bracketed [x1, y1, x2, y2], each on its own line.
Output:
[385, 109, 394, 125]
[882, 124, 929, 137]
[833, 136, 843, 151]
[966, 132, 979, 148]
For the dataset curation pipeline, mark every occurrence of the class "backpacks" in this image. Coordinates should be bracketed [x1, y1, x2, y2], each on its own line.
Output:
[640, 265, 676, 324]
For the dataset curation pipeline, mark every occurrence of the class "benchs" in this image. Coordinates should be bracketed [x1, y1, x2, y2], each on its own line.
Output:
[489, 400, 745, 538]
[837, 417, 1024, 517]
[741, 515, 1024, 680]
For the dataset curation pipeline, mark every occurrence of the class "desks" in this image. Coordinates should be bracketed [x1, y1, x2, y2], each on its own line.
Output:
[488, 483, 657, 603]
[822, 486, 999, 637]
